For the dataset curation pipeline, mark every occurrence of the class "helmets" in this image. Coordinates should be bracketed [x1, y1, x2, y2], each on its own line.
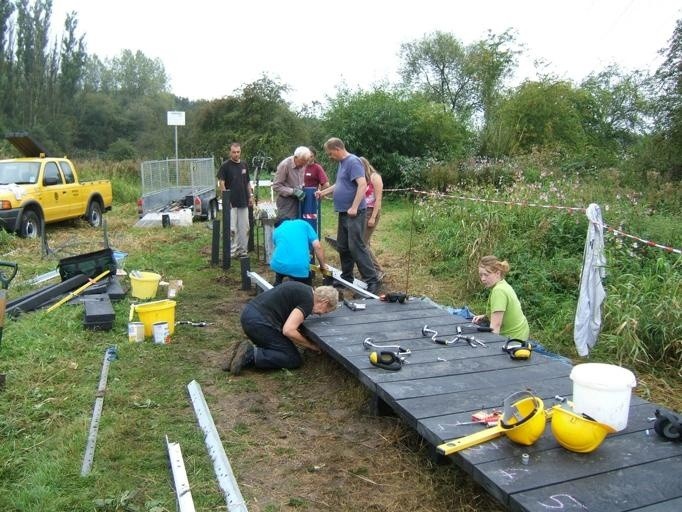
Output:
[501, 391, 547, 446]
[551, 400, 618, 452]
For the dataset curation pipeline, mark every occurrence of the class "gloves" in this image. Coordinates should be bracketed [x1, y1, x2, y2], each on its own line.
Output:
[292, 188, 305, 201]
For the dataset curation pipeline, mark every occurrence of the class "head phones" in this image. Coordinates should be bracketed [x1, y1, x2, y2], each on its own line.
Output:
[655, 408, 682, 443]
[369, 349, 402, 370]
[388, 292, 408, 303]
[504, 339, 532, 359]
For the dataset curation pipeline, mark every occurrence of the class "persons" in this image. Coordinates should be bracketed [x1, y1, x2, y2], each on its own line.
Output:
[216, 143, 256, 258]
[304, 145, 330, 191]
[315, 136, 381, 294]
[223, 280, 339, 375]
[269, 217, 329, 287]
[472, 255, 530, 344]
[359, 156, 387, 280]
[272, 146, 311, 223]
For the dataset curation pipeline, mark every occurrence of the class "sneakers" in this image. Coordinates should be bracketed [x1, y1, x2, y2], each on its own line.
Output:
[334, 273, 385, 292]
[222, 339, 238, 371]
[231, 341, 255, 376]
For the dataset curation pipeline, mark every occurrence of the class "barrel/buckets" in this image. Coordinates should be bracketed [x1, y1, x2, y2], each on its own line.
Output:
[568, 361, 637, 432]
[129, 271, 161, 299]
[133, 298, 177, 336]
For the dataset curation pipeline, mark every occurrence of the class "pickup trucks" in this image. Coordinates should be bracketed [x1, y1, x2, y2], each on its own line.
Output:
[0, 153, 113, 239]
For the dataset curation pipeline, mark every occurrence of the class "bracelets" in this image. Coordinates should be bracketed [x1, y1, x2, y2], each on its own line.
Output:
[248, 193, 253, 196]
